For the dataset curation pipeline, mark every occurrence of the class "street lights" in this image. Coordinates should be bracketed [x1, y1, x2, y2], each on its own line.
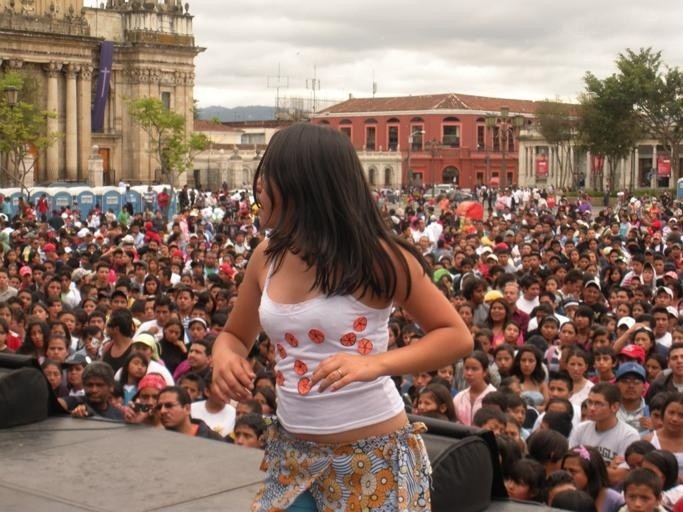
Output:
[483, 105, 524, 194]
[406, 129, 426, 183]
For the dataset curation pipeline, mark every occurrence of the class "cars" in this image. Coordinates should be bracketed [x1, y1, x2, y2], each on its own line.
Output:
[434, 183, 453, 193]
[423, 188, 443, 201]
